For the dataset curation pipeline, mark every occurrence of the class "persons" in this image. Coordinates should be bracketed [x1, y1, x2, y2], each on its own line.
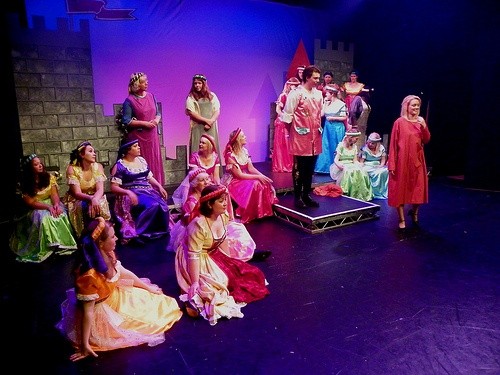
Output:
[388, 95, 430, 232]
[221, 128, 280, 224]
[110, 138, 170, 246]
[279, 65, 324, 208]
[185, 74, 223, 180]
[329, 129, 374, 201]
[175, 185, 270, 325]
[269, 77, 301, 192]
[296, 65, 372, 153]
[54, 217, 183, 361]
[8, 154, 78, 263]
[359, 132, 389, 199]
[166, 167, 212, 253]
[122, 72, 166, 192]
[61, 141, 111, 238]
[187, 134, 221, 185]
[314, 86, 349, 174]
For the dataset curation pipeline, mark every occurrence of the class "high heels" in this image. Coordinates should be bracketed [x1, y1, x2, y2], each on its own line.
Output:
[407, 210, 419, 226]
[398, 219, 406, 233]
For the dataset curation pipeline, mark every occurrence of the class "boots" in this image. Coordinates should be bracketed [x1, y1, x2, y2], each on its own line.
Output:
[303, 182, 319, 207]
[293, 182, 307, 208]
